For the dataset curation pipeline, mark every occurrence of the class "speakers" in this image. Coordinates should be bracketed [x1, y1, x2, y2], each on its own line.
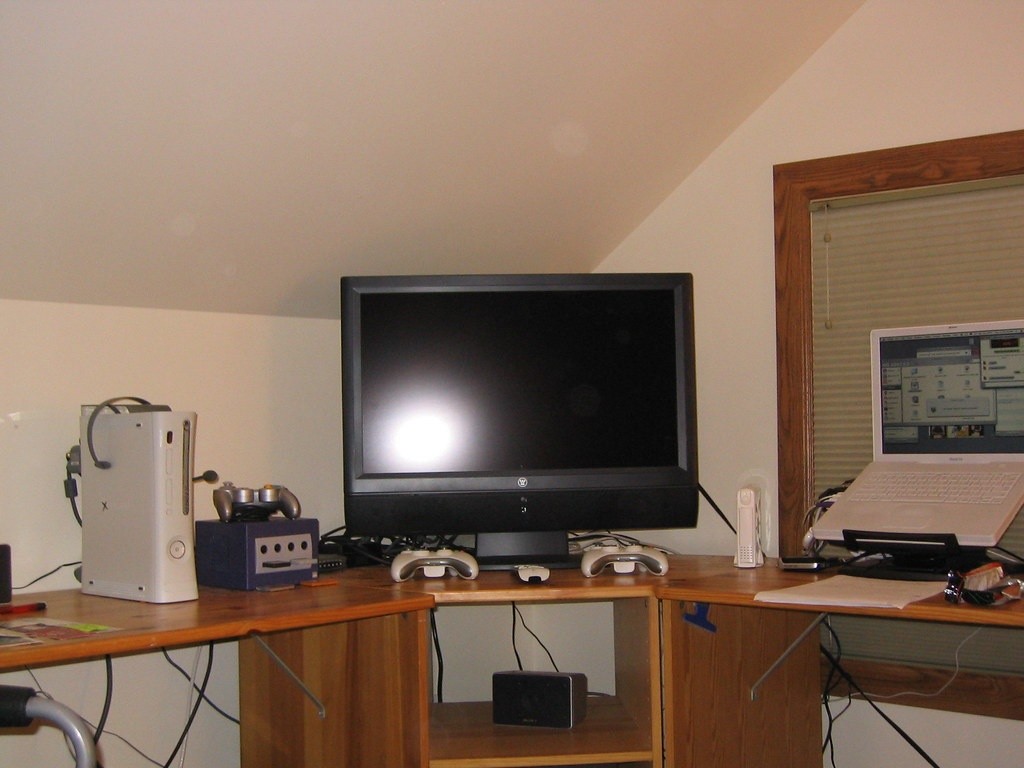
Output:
[492, 670, 588, 729]
[78, 412, 198, 604]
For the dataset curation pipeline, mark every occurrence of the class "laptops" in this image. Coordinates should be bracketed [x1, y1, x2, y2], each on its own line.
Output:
[813, 320, 1024, 546]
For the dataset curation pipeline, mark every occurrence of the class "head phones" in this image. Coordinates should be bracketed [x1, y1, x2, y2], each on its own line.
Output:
[88, 398, 153, 470]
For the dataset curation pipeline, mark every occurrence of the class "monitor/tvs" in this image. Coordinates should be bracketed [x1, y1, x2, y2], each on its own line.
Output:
[340, 276, 699, 569]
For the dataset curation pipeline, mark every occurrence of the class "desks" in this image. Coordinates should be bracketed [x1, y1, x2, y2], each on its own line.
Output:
[0, 584, 436, 768]
[657, 564, 1024, 768]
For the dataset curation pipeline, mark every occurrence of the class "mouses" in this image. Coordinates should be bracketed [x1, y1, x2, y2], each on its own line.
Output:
[514, 564, 549, 584]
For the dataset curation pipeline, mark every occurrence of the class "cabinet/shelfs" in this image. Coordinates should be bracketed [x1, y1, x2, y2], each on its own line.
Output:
[238, 555, 823, 768]
